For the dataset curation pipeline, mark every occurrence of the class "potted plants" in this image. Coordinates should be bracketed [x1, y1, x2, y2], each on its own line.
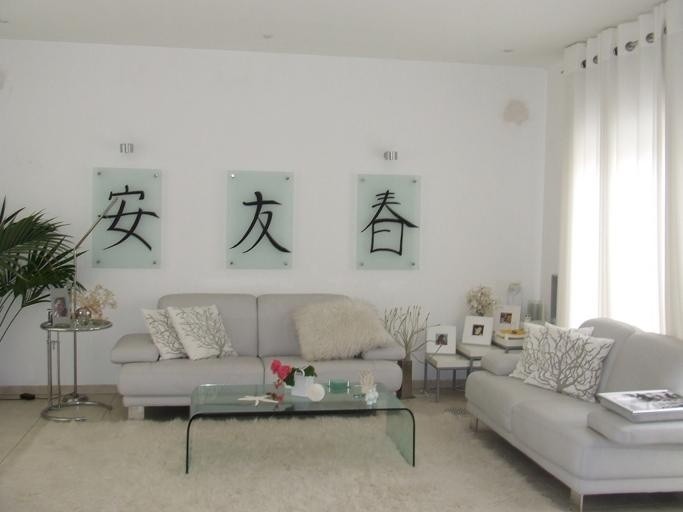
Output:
[380, 304, 443, 399]
[67, 285, 114, 324]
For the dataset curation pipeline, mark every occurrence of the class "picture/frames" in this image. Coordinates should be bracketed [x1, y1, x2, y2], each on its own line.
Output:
[423, 325, 455, 355]
[49, 291, 71, 326]
[461, 314, 493, 349]
[492, 303, 521, 332]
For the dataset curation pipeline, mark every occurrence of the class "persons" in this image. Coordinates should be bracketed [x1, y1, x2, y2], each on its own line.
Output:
[472, 325, 483, 336]
[53, 298, 68, 318]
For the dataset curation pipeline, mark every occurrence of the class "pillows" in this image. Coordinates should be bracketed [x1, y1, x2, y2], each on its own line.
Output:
[290, 299, 375, 362]
[141, 307, 187, 361]
[520, 321, 615, 404]
[368, 325, 395, 348]
[509, 321, 596, 381]
[165, 304, 239, 360]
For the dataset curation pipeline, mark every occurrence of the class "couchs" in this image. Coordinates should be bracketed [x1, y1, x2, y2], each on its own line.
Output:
[109, 293, 407, 421]
[463, 316, 682, 512]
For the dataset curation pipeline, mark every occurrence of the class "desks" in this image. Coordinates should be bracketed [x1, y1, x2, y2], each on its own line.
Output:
[39, 316, 114, 425]
[422, 337, 524, 404]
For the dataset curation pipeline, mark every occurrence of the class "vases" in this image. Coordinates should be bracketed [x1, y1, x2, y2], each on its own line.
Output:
[291, 375, 312, 396]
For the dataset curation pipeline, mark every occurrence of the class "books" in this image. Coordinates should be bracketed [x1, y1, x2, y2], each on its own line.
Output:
[594, 389, 683, 422]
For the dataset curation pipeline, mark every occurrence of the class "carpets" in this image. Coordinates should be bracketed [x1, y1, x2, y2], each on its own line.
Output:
[1, 409, 566, 512]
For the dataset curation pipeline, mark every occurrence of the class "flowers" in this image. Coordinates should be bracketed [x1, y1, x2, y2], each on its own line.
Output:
[271, 357, 316, 383]
[270, 391, 295, 411]
[465, 286, 500, 315]
